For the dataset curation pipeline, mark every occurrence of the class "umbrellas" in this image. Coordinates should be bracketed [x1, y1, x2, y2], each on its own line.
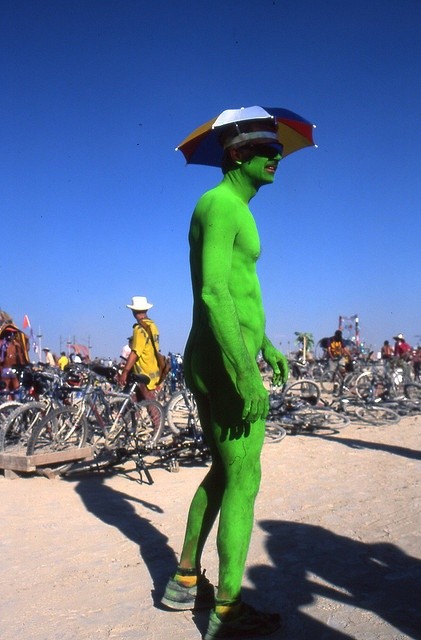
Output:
[174, 106, 319, 166]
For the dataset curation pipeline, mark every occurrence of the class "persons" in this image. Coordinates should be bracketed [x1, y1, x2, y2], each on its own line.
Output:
[120, 336, 133, 367]
[168, 352, 184, 375]
[117, 296, 163, 441]
[0, 310, 30, 438]
[328, 331, 358, 397]
[153, 119, 289, 640]
[58, 352, 69, 370]
[69, 352, 116, 371]
[382, 341, 394, 369]
[43, 348, 56, 367]
[393, 333, 412, 365]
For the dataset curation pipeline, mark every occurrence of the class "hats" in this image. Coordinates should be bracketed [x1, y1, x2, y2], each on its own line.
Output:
[126, 297, 153, 310]
[393, 333, 405, 342]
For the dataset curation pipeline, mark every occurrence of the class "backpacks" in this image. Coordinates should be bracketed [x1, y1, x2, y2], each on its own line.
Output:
[331, 338, 344, 357]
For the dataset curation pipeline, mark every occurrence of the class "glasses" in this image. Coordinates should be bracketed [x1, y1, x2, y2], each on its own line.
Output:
[238, 140, 284, 156]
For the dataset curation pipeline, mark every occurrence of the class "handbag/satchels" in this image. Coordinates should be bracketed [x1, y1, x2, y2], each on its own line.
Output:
[133, 320, 171, 385]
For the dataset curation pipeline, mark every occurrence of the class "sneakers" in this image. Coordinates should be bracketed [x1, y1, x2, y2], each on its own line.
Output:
[161, 569, 219, 611]
[204, 603, 283, 638]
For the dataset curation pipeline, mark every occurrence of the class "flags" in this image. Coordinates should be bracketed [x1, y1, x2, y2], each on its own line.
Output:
[24, 315, 28, 329]
